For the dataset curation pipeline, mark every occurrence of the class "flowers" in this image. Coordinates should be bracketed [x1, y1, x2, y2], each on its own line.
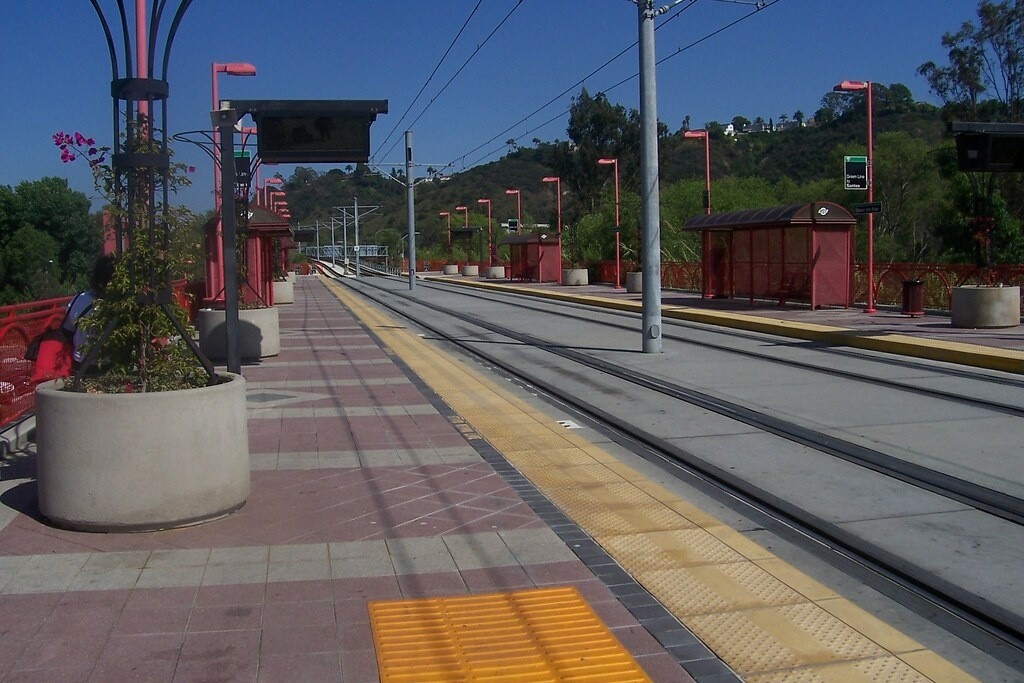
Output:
[52, 111, 214, 394]
[211, 188, 259, 308]
[965, 217, 998, 286]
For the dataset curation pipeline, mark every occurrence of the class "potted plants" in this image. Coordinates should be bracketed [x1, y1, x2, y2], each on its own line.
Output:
[442, 223, 643, 294]
[270, 267, 298, 302]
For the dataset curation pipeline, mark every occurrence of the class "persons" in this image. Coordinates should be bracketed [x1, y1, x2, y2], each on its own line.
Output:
[59, 256, 115, 369]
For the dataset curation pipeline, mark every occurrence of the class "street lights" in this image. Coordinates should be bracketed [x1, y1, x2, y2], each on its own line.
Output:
[212, 62, 256, 308]
[597, 159, 620, 289]
[440, 213, 451, 246]
[241, 126, 256, 156]
[478, 199, 492, 266]
[264, 179, 292, 306]
[506, 189, 522, 274]
[684, 129, 711, 299]
[402, 233, 420, 272]
[834, 81, 874, 311]
[337, 241, 355, 256]
[375, 229, 389, 256]
[543, 177, 562, 284]
[455, 206, 468, 228]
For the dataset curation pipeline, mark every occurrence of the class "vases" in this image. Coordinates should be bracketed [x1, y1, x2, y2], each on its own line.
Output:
[32, 372, 250, 533]
[949, 287, 1020, 326]
[198, 307, 281, 364]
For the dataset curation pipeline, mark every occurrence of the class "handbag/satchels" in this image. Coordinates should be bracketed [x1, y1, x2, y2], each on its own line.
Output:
[25, 325, 73, 383]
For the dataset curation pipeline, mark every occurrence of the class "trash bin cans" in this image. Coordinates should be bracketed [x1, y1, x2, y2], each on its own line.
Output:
[505, 266, 511, 278]
[901, 280, 925, 317]
